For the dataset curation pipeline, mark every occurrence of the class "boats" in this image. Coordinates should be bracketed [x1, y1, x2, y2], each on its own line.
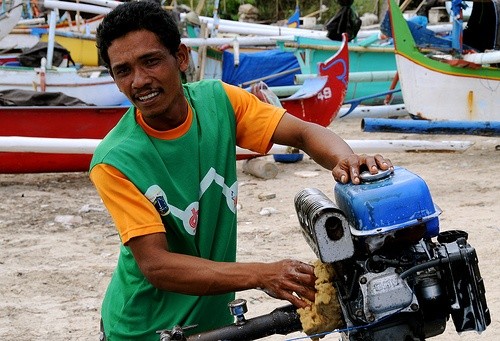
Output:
[1, 1, 399, 108]
[388, 0, 500, 131]
[0, 31, 352, 174]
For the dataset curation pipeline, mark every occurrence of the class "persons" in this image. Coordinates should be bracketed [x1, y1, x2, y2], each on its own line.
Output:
[88, 0, 395, 340]
